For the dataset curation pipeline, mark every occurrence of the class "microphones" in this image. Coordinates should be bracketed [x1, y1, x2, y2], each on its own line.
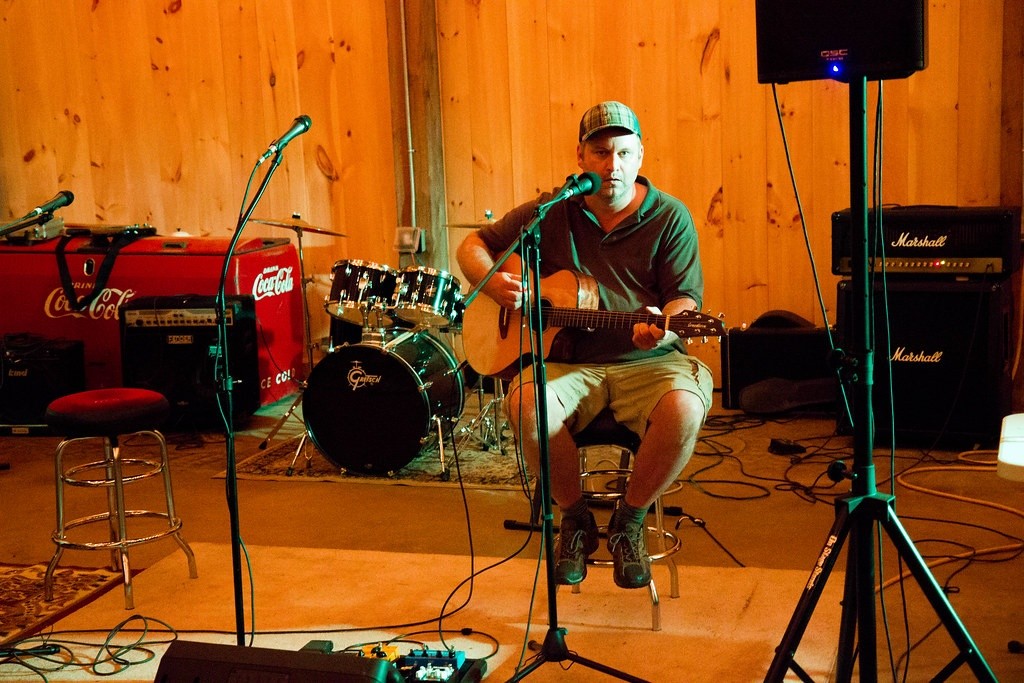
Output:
[562, 172, 602, 200]
[257, 114, 312, 165]
[27, 190, 75, 217]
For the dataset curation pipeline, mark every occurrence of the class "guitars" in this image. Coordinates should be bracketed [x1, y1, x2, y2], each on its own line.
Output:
[462, 251, 727, 379]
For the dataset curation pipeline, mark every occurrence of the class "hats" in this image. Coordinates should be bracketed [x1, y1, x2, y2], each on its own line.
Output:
[579, 100, 642, 145]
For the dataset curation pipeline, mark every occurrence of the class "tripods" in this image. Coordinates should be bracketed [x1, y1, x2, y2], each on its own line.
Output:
[761, 73, 1002, 683]
[438, 377, 508, 482]
[458, 171, 651, 683]
[443, 373, 506, 446]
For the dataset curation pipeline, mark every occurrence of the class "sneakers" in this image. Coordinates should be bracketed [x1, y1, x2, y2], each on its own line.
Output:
[554, 512, 599, 584]
[606, 511, 651, 588]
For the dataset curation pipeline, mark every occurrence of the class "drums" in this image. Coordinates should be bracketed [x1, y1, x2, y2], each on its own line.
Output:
[303, 326, 464, 476]
[325, 258, 400, 324]
[388, 265, 459, 326]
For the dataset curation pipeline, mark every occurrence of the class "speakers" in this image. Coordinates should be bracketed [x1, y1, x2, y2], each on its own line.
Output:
[0, 340, 85, 440]
[155, 639, 408, 683]
[117, 294, 261, 434]
[755, 0, 929, 85]
[837, 277, 1017, 451]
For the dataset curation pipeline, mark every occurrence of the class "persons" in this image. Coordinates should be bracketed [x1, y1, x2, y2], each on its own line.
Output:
[454, 101, 715, 589]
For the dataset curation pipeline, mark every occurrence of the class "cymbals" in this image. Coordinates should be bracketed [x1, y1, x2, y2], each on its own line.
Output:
[250, 218, 344, 245]
[439, 220, 495, 231]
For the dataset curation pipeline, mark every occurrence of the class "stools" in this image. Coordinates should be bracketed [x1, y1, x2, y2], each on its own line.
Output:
[44, 388, 199, 609]
[552, 406, 683, 631]
